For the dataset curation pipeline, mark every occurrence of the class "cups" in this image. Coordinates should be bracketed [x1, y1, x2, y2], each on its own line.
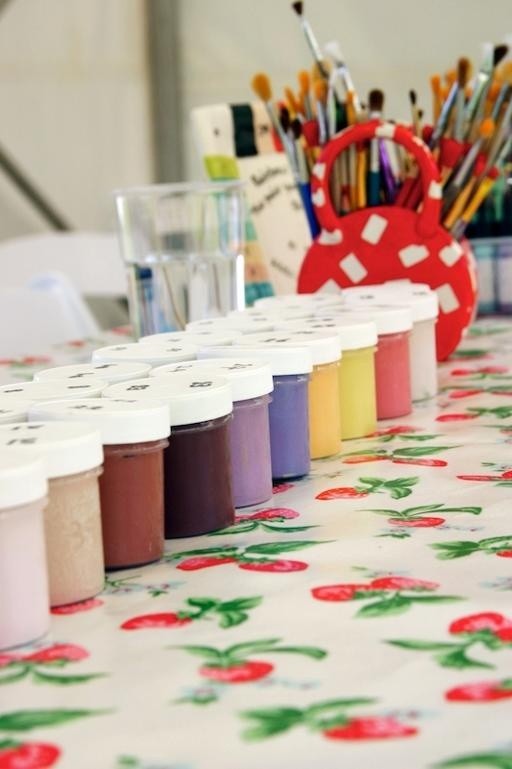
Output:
[118, 184, 245, 340]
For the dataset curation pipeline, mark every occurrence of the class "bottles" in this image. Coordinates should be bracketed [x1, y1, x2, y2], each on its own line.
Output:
[33, 363, 151, 395]
[150, 359, 273, 508]
[138, 331, 242, 359]
[0, 380, 67, 424]
[337, 306, 412, 419]
[103, 373, 235, 539]
[344, 280, 439, 401]
[91, 343, 174, 366]
[253, 294, 347, 318]
[30, 396, 172, 571]
[185, 317, 273, 334]
[1, 460, 50, 650]
[0, 419, 105, 607]
[197, 344, 309, 480]
[231, 329, 342, 460]
[276, 315, 377, 440]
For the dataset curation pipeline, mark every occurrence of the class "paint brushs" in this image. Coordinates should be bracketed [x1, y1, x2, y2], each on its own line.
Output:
[250, 0, 512, 244]
